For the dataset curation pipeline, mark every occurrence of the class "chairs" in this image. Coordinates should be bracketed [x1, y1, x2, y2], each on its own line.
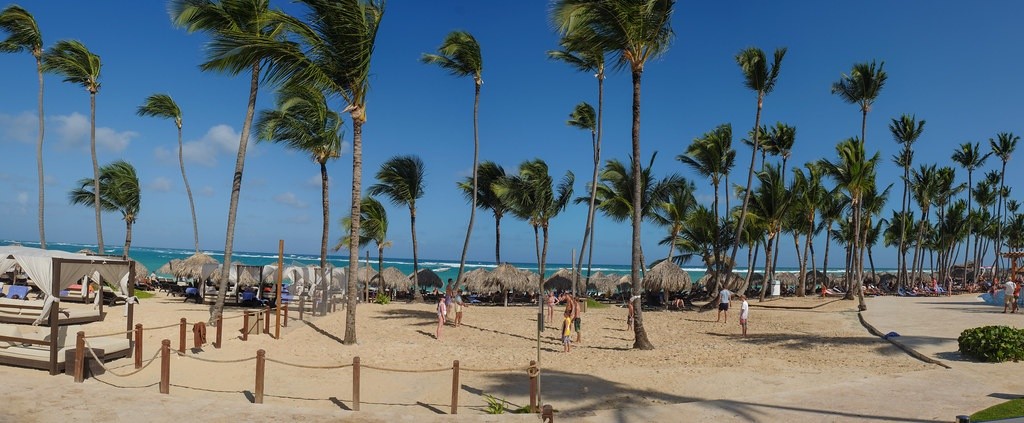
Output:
[156, 277, 203, 304]
[241, 291, 264, 309]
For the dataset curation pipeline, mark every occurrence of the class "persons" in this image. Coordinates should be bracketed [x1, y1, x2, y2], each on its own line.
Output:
[626, 301, 633, 331]
[545, 291, 559, 322]
[561, 293, 581, 353]
[436, 294, 447, 341]
[740, 295, 748, 336]
[455, 289, 468, 327]
[433, 286, 440, 303]
[863, 273, 1000, 296]
[151, 271, 162, 289]
[1001, 273, 1024, 284]
[672, 296, 686, 310]
[1001, 276, 1021, 313]
[821, 282, 826, 299]
[717, 284, 732, 323]
[445, 278, 456, 317]
